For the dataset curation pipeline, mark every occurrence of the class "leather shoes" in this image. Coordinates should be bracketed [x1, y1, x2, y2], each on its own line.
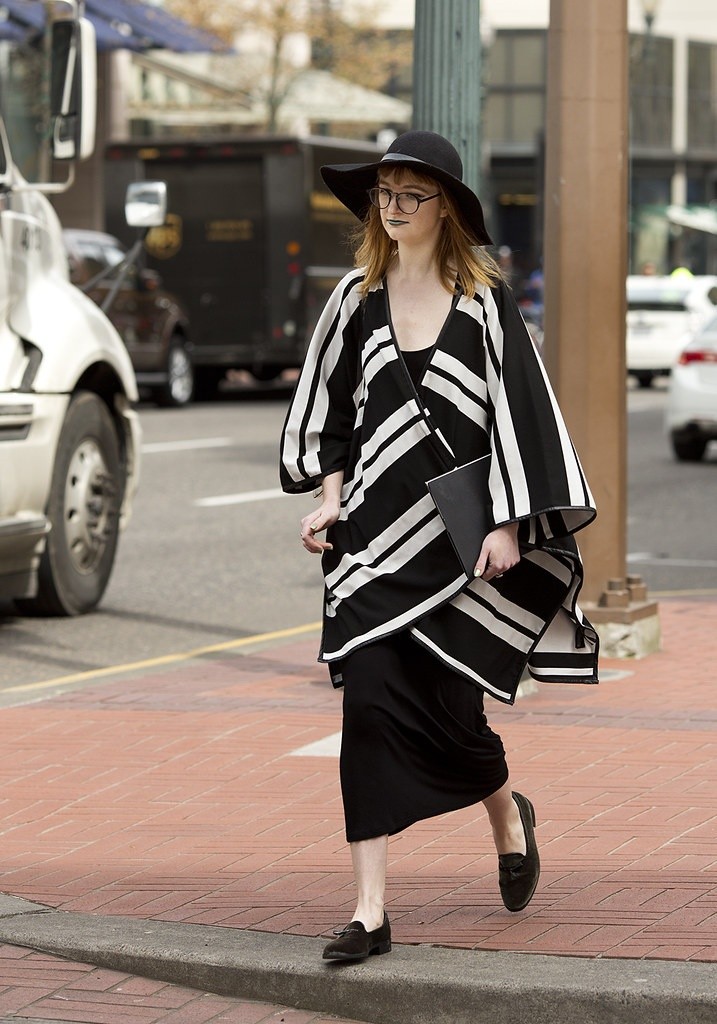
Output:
[323, 911, 393, 960]
[496, 790, 540, 911]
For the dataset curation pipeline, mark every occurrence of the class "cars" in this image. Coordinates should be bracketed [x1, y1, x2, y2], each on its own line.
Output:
[625, 274, 717, 391]
[666, 314, 717, 464]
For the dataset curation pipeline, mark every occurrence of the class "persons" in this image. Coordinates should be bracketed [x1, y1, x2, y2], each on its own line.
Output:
[274, 129, 599, 959]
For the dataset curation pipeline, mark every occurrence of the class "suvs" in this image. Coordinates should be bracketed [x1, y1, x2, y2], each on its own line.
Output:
[60, 231, 194, 406]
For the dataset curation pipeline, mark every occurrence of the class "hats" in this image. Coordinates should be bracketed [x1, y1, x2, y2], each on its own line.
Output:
[318, 130, 496, 247]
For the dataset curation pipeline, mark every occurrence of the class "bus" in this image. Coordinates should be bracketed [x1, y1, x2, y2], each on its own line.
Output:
[0, 0, 145, 616]
[103, 134, 392, 412]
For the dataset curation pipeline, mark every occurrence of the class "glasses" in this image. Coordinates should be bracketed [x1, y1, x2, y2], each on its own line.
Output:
[366, 187, 442, 216]
